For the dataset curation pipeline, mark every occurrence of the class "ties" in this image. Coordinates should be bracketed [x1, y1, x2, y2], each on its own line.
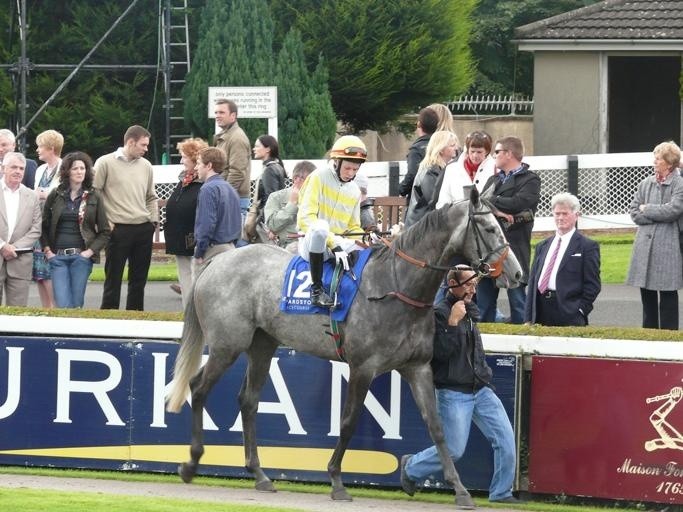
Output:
[538, 238, 562, 294]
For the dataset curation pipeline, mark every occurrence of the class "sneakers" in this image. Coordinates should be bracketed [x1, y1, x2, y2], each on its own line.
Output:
[489, 496, 527, 504]
[171, 284, 180, 293]
[400, 454, 415, 496]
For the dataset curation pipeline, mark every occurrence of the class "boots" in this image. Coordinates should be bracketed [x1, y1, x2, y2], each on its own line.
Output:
[309, 252, 334, 308]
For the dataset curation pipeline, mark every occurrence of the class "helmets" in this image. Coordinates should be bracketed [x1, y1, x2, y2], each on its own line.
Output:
[330, 135, 368, 162]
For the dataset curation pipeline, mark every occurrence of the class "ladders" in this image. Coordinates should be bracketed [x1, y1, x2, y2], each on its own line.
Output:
[160, 0, 191, 163]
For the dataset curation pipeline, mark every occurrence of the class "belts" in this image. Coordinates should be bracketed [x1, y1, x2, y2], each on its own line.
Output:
[545, 291, 551, 298]
[58, 247, 82, 255]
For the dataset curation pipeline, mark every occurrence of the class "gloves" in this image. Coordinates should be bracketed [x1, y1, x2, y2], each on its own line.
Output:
[331, 246, 353, 271]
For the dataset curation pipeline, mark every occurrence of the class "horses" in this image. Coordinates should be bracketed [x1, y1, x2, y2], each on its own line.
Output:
[170, 182, 523, 507]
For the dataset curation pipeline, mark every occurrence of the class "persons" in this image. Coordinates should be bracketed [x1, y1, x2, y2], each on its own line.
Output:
[0, 152, 44, 298]
[427, 103, 453, 136]
[476, 137, 539, 324]
[164, 139, 207, 313]
[400, 264, 523, 503]
[525, 191, 602, 327]
[405, 133, 458, 230]
[252, 134, 287, 244]
[263, 161, 313, 245]
[33, 130, 64, 308]
[296, 134, 366, 306]
[399, 111, 440, 194]
[436, 132, 494, 209]
[212, 99, 251, 248]
[628, 140, 683, 331]
[354, 174, 368, 225]
[192, 147, 242, 280]
[93, 125, 152, 311]
[0, 129, 37, 190]
[39, 152, 111, 306]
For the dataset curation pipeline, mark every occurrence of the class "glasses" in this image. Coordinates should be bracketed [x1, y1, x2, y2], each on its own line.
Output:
[495, 150, 507, 154]
[345, 147, 368, 158]
